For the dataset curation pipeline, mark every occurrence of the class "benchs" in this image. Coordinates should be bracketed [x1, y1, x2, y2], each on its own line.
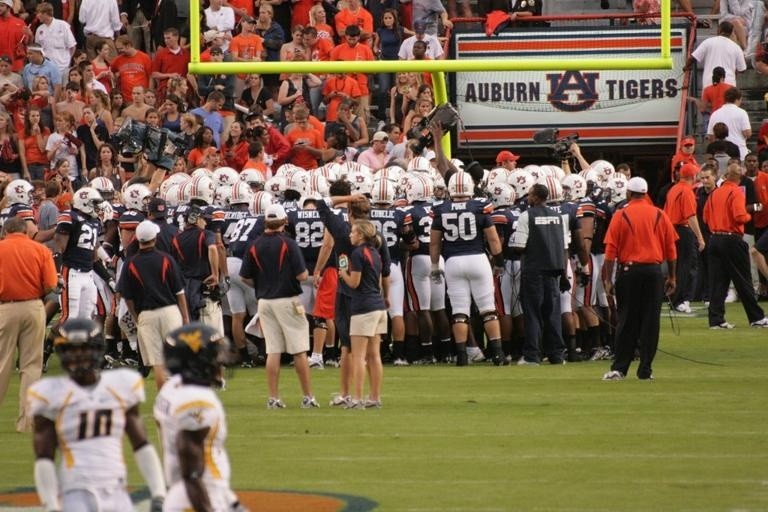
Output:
[170, 1, 192, 34]
[364, 0, 766, 178]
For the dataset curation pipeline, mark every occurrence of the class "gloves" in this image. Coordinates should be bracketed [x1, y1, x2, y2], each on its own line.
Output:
[429, 269, 445, 285]
[575, 261, 591, 287]
[560, 274, 571, 293]
[492, 265, 505, 277]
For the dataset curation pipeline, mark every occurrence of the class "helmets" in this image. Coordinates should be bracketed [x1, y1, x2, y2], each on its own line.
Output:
[50, 318, 107, 386]
[159, 156, 434, 216]
[161, 323, 231, 390]
[72, 176, 115, 223]
[449, 172, 475, 198]
[4, 179, 35, 207]
[448, 157, 465, 171]
[121, 177, 153, 213]
[486, 160, 629, 210]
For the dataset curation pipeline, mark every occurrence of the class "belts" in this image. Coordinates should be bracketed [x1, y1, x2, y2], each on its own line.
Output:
[708, 231, 743, 239]
[678, 224, 689, 228]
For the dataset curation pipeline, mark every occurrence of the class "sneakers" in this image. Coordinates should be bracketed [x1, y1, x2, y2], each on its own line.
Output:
[601, 370, 625, 382]
[241, 344, 614, 369]
[329, 394, 382, 411]
[301, 395, 320, 408]
[268, 398, 286, 409]
[100, 338, 151, 377]
[725, 291, 737, 303]
[709, 322, 736, 330]
[750, 316, 768, 328]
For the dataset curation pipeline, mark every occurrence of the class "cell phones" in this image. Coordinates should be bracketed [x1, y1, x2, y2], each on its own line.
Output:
[297, 141, 305, 144]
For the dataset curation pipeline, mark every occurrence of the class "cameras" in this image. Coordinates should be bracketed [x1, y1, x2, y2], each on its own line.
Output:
[61, 137, 68, 142]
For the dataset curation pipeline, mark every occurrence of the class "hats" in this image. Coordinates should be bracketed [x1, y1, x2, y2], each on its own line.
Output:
[245, 111, 262, 122]
[679, 135, 697, 147]
[265, 203, 287, 222]
[149, 199, 168, 219]
[674, 160, 701, 178]
[240, 16, 256, 25]
[135, 219, 160, 242]
[496, 150, 520, 167]
[626, 176, 649, 194]
[370, 132, 388, 146]
[1, 0, 13, 9]
